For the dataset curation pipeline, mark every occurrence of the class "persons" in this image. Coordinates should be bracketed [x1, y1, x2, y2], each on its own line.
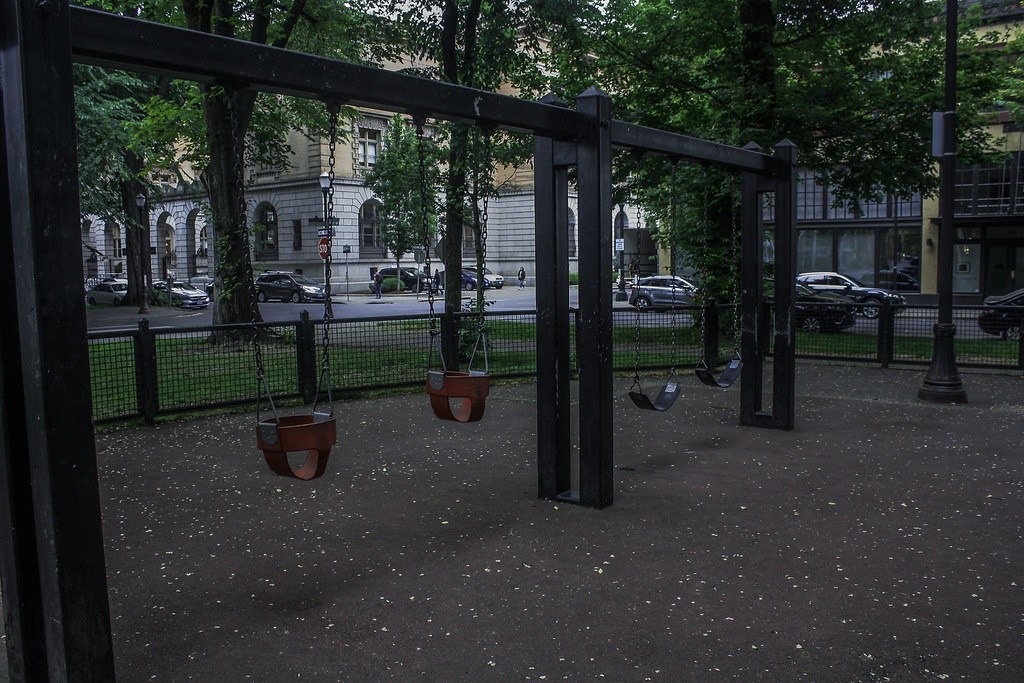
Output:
[434, 269, 443, 296]
[373, 269, 381, 299]
[517, 267, 525, 291]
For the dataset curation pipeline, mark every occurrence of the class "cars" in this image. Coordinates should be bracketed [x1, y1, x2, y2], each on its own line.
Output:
[87, 280, 128, 307]
[462, 264, 506, 289]
[628, 273, 718, 313]
[373, 267, 439, 293]
[762, 277, 857, 336]
[437, 268, 491, 291]
[977, 287, 1024, 341]
[151, 280, 210, 310]
[853, 268, 920, 293]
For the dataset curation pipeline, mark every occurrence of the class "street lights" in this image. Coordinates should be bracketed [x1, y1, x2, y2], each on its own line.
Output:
[318, 170, 335, 318]
[614, 184, 628, 302]
[134, 191, 153, 314]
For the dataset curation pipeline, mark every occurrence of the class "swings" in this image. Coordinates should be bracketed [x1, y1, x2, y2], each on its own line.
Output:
[406, 107, 499, 423]
[694, 158, 745, 388]
[207, 74, 352, 482]
[624, 146, 684, 413]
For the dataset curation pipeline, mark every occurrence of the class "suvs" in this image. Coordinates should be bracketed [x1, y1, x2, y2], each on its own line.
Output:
[253, 271, 327, 304]
[795, 270, 907, 319]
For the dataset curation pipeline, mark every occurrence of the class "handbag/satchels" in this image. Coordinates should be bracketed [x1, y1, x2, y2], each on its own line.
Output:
[523, 280, 527, 285]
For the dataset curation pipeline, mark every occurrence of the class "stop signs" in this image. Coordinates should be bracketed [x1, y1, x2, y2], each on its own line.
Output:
[318, 237, 331, 260]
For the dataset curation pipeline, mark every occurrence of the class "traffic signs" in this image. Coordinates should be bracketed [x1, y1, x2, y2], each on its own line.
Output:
[411, 244, 426, 251]
[317, 227, 336, 237]
